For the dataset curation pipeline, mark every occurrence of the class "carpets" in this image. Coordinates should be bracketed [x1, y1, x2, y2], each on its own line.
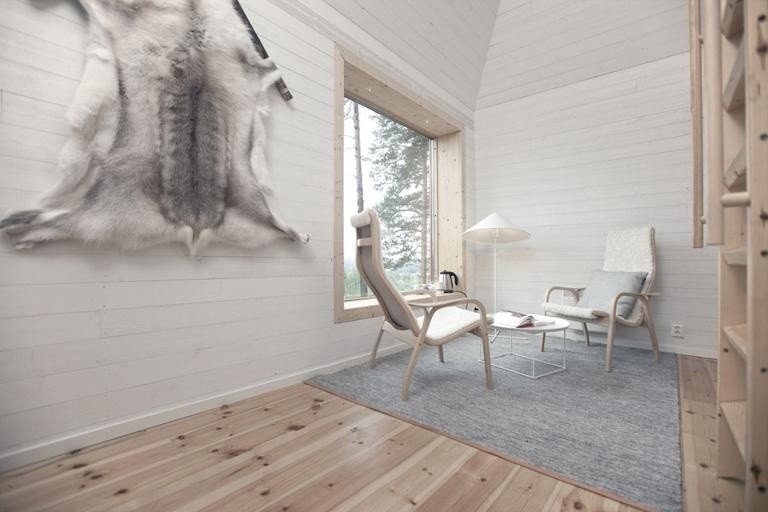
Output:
[301, 318, 684, 510]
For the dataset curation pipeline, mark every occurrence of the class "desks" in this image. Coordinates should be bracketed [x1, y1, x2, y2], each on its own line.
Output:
[478, 310, 571, 380]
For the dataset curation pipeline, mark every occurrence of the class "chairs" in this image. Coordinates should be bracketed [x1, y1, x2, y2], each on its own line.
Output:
[538, 223, 661, 375]
[351, 209, 496, 401]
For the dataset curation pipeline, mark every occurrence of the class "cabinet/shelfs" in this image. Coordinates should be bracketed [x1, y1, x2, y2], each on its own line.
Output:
[714, 1, 768, 510]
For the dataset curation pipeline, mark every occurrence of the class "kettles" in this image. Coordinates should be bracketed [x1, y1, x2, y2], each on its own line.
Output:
[439, 270, 459, 294]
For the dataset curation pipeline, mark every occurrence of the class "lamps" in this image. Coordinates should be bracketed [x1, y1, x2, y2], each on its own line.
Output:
[460, 210, 535, 309]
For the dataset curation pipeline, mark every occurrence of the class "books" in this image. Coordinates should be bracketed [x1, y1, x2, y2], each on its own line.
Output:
[493, 309, 557, 328]
